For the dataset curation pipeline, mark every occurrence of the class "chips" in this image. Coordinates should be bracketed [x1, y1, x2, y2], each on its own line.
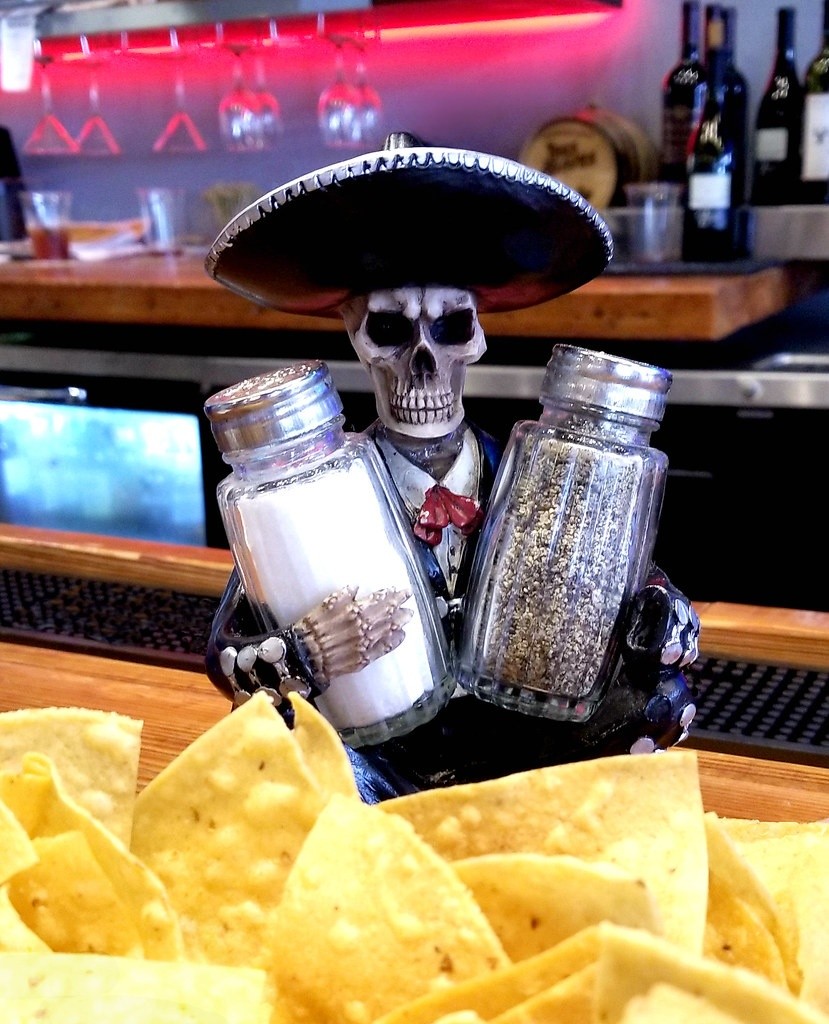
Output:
[1, 690, 827, 1024]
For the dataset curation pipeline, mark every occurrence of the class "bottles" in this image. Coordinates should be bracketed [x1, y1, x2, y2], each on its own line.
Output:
[454, 338, 676, 727]
[206, 365, 455, 748]
[661, 0, 829, 267]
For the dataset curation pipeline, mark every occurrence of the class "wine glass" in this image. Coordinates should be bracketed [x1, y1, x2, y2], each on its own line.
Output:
[248, 40, 284, 144]
[349, 29, 380, 149]
[219, 42, 265, 153]
[321, 28, 364, 150]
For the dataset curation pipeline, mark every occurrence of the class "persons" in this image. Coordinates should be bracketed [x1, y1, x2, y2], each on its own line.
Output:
[204, 285, 703, 801]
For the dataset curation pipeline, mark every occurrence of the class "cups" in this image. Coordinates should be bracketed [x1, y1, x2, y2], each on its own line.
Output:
[135, 182, 188, 258]
[624, 181, 684, 263]
[18, 186, 73, 260]
